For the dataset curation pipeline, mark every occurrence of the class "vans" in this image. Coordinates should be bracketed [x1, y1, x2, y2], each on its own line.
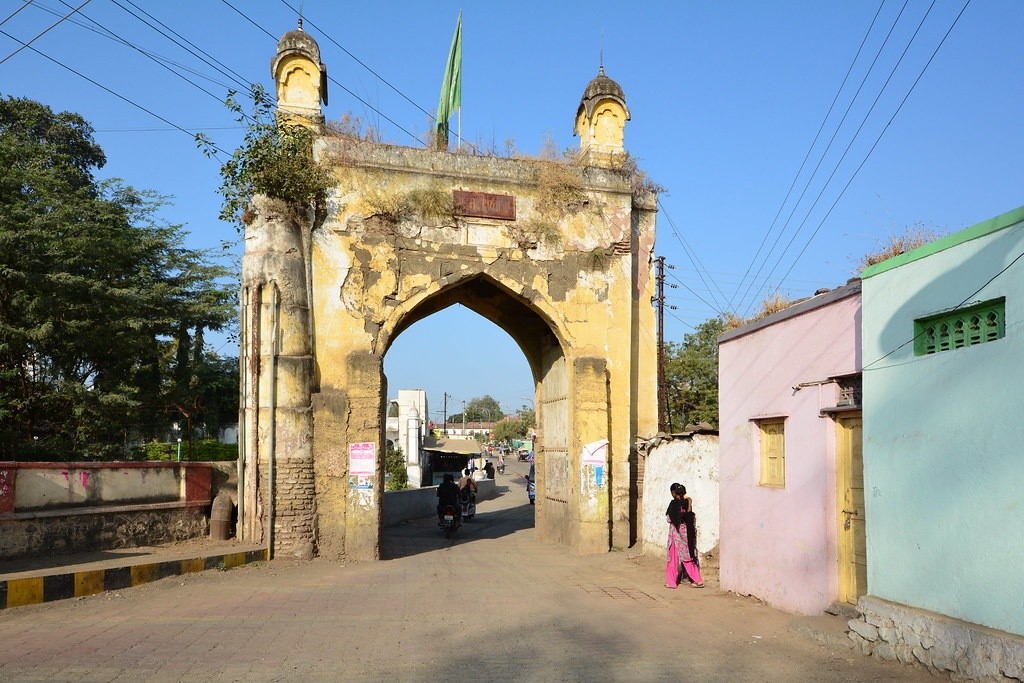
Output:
[524, 465, 535, 504]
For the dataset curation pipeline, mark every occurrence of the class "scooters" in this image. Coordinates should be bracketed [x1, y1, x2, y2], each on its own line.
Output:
[460, 490, 476, 522]
[498, 463, 504, 476]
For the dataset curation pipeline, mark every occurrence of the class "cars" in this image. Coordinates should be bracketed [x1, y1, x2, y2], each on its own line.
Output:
[517, 449, 530, 462]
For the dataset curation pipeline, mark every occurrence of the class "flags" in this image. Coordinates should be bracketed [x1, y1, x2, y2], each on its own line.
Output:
[435, 12, 463, 138]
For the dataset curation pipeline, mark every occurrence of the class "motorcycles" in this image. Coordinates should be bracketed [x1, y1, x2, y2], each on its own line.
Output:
[435, 487, 462, 540]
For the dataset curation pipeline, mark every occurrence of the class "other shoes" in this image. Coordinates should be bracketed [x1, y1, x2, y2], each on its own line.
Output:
[664, 584, 677, 589]
[690, 582, 705, 588]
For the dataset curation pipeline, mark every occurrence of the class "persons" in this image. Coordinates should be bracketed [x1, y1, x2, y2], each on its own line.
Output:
[481, 439, 534, 479]
[665, 483, 704, 589]
[437, 469, 478, 527]
